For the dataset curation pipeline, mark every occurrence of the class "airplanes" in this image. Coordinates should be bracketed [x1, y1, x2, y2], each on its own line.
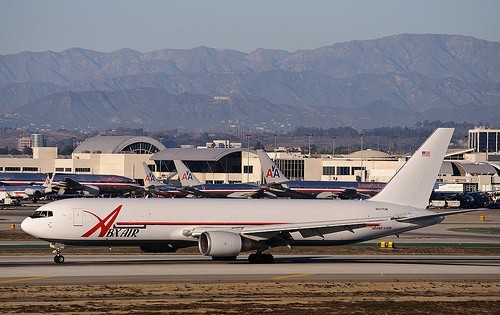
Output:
[21, 126, 490, 264]
[0, 156, 392, 211]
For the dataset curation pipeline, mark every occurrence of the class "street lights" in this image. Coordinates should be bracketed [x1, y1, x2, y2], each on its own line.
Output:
[306, 134, 314, 159]
[332, 136, 337, 158]
[274, 133, 277, 153]
[359, 133, 365, 182]
[156, 137, 164, 179]
[245, 133, 252, 183]
